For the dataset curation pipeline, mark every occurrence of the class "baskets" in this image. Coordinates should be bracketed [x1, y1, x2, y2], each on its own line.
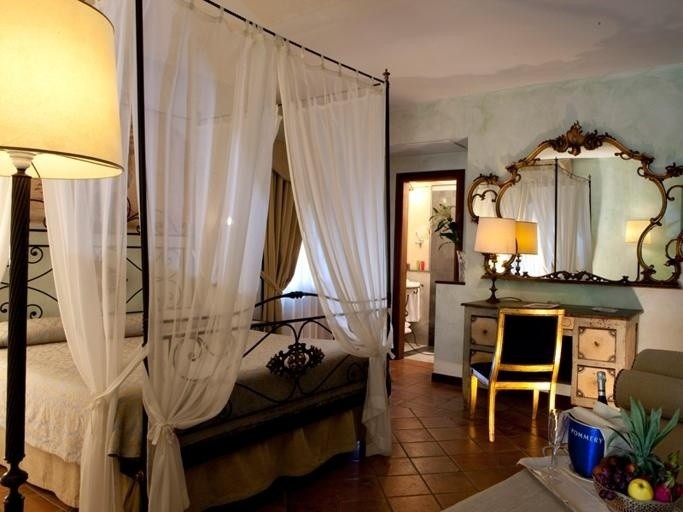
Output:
[591, 474, 682, 512]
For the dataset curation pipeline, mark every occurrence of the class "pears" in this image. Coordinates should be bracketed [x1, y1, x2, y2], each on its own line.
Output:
[627, 478, 654, 501]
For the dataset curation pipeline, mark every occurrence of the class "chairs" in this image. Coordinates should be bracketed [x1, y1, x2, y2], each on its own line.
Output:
[466, 307, 566, 444]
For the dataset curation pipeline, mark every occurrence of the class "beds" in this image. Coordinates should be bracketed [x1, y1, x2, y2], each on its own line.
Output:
[1, 0, 397, 511]
[472, 154, 593, 281]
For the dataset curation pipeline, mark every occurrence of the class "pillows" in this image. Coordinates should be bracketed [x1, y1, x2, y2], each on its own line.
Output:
[0, 313, 143, 351]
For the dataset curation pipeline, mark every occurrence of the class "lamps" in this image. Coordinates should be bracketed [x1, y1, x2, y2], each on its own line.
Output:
[511, 217, 539, 278]
[472, 216, 516, 304]
[0, 0, 127, 512]
[623, 219, 653, 281]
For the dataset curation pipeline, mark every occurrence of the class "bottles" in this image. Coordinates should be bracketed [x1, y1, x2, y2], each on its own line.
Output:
[595, 371, 608, 404]
[415, 259, 424, 271]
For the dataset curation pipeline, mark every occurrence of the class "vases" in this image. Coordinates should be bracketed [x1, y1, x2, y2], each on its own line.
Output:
[454, 249, 465, 284]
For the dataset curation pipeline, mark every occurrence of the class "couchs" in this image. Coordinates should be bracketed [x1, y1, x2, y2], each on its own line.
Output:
[612, 347, 682, 489]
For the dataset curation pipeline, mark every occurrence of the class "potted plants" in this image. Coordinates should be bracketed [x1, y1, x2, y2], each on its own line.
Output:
[427, 201, 460, 250]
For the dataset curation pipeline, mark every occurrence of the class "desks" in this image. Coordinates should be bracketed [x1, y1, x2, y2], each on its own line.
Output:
[435, 468, 608, 512]
[457, 296, 644, 410]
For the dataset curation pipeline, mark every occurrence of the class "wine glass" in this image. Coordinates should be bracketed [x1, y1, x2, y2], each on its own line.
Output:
[541, 408, 567, 477]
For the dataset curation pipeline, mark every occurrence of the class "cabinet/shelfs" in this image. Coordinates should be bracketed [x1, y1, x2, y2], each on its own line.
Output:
[431, 278, 466, 386]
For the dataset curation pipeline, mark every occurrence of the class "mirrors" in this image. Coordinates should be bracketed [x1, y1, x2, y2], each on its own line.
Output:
[466, 120, 682, 292]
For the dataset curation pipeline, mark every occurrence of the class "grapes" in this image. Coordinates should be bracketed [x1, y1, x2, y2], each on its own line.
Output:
[593, 454, 633, 500]
